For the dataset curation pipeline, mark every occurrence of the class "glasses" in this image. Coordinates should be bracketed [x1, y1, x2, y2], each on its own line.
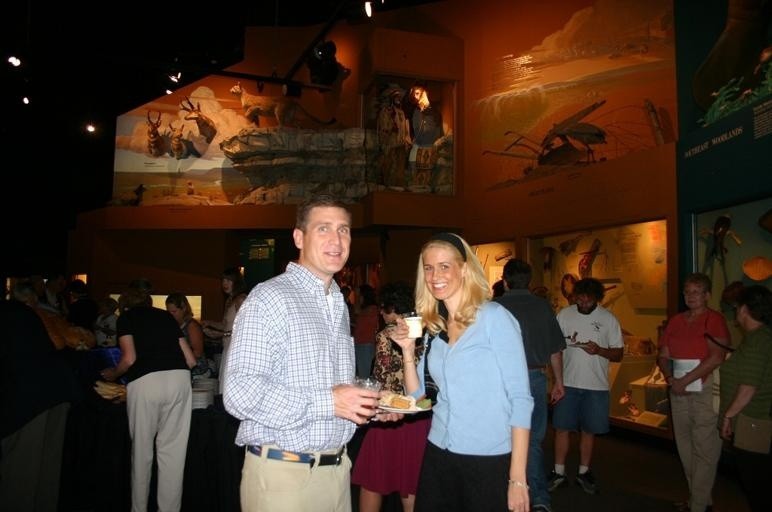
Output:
[575, 299, 594, 309]
[683, 290, 702, 296]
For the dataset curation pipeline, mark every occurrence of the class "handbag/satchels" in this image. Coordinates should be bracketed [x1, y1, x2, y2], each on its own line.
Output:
[734, 412, 772, 454]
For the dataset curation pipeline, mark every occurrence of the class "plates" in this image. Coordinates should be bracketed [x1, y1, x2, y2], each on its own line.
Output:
[189, 378, 219, 410]
[376, 397, 432, 415]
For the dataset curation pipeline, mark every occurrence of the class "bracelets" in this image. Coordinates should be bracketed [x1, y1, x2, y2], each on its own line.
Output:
[665, 375, 671, 384]
[508, 479, 530, 489]
[723, 414, 733, 421]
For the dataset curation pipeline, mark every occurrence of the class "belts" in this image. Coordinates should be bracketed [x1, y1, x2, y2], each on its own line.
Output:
[246, 445, 345, 468]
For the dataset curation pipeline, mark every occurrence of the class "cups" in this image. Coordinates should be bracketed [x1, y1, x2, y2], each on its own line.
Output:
[404, 317, 423, 339]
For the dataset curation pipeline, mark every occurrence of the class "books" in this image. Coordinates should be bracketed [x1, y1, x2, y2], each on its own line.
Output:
[670, 358, 703, 392]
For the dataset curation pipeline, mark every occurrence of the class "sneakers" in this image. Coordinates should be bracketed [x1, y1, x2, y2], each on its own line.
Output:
[547, 472, 567, 493]
[576, 468, 599, 496]
[532, 503, 553, 511]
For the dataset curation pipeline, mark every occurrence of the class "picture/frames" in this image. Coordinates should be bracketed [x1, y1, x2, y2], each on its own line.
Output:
[686, 191, 771, 351]
[518, 208, 682, 442]
[461, 233, 525, 292]
[357, 66, 464, 200]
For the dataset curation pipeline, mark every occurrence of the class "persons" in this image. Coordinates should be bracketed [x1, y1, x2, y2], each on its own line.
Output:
[408, 85, 445, 192]
[494, 259, 567, 512]
[387, 231, 535, 512]
[350, 281, 438, 512]
[0, 258, 266, 512]
[223, 195, 380, 512]
[374, 83, 411, 192]
[715, 284, 771, 512]
[351, 283, 379, 380]
[658, 273, 731, 512]
[546, 277, 624, 494]
